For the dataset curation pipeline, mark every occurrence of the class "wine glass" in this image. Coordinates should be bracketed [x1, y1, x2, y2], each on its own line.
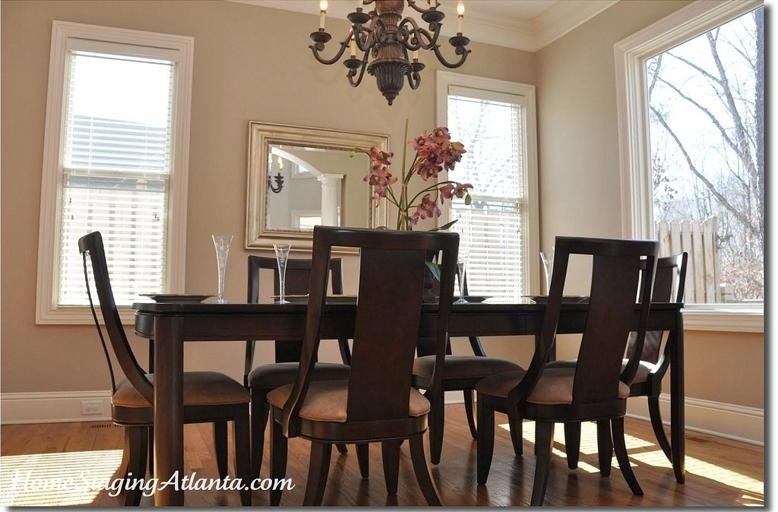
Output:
[212, 233, 232, 304]
[539, 251, 554, 296]
[272, 241, 293, 303]
[453, 242, 472, 305]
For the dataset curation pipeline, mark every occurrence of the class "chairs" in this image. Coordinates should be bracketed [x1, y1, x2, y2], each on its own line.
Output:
[265, 222, 460, 510]
[469, 230, 660, 509]
[76, 230, 259, 510]
[243, 249, 359, 487]
[540, 250, 690, 498]
[406, 260, 532, 465]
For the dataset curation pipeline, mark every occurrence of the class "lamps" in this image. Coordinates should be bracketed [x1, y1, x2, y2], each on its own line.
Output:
[268, 147, 287, 195]
[304, 0, 472, 111]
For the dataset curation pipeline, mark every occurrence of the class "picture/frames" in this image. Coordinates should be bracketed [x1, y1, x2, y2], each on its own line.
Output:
[244, 118, 391, 256]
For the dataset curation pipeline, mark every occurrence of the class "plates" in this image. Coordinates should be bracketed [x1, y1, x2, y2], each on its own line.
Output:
[270, 294, 357, 303]
[453, 296, 494, 303]
[138, 294, 215, 304]
[524, 295, 586, 303]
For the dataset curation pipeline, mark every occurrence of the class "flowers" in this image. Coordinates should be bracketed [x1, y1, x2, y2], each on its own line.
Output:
[345, 116, 478, 285]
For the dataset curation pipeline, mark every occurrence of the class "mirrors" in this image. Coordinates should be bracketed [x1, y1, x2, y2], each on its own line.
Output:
[265, 141, 369, 231]
